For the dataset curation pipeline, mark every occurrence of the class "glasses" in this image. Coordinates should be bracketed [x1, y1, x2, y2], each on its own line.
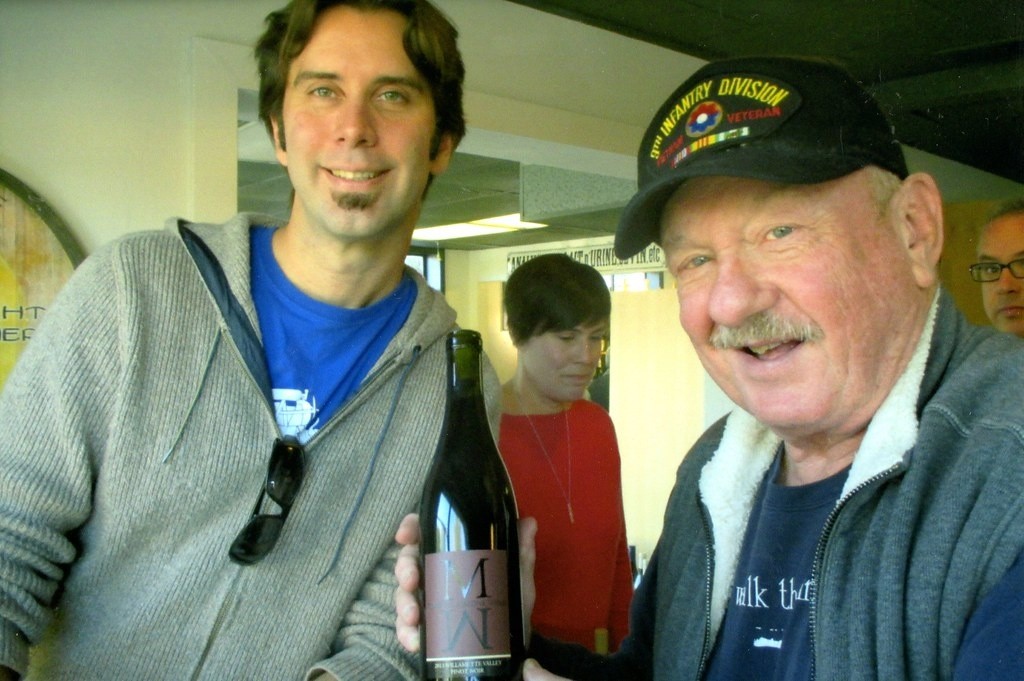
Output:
[227, 435, 307, 566]
[968, 258, 1024, 282]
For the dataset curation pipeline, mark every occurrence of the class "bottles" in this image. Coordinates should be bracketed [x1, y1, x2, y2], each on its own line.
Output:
[415, 329, 526, 681]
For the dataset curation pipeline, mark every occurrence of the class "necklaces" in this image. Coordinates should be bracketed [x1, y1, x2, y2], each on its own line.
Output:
[506, 380, 575, 527]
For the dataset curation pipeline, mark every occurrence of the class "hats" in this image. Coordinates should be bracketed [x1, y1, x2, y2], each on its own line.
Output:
[614, 55, 908, 259]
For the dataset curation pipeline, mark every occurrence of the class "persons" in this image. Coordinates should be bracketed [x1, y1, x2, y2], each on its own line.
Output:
[0, 0, 506, 681]
[394, 49, 1024, 681]
